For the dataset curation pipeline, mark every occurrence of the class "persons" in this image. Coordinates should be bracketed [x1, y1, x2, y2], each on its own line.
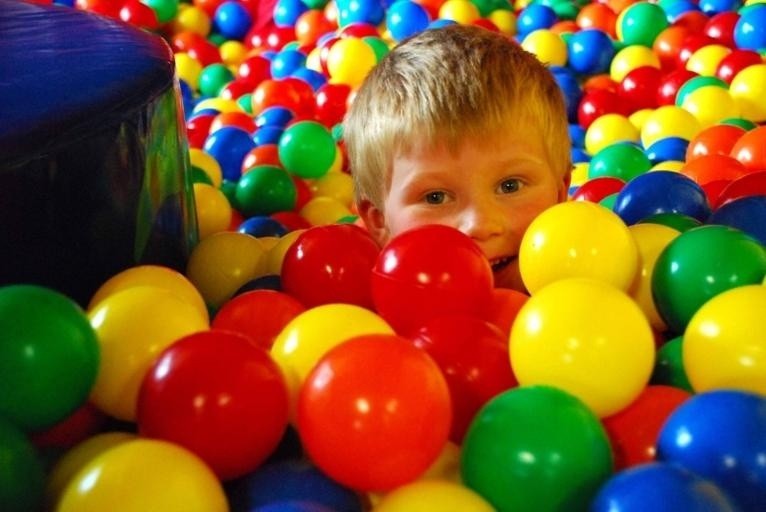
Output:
[342, 25, 575, 272]
